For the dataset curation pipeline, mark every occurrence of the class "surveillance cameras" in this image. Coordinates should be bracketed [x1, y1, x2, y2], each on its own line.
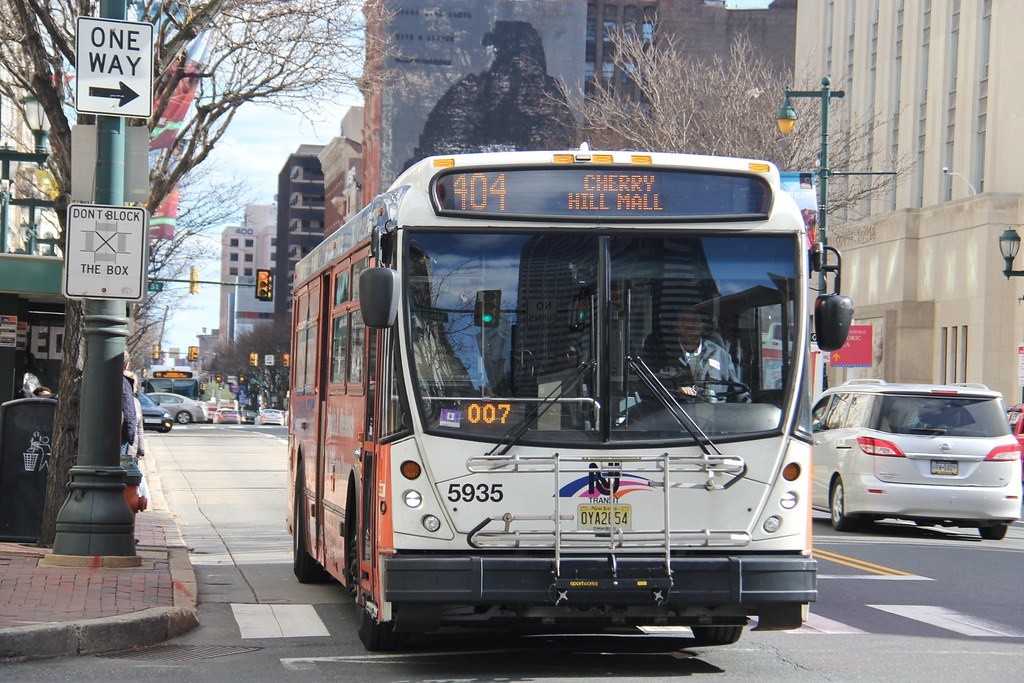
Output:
[943, 167, 947, 173]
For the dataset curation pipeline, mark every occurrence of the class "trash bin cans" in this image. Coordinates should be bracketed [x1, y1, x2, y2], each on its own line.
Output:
[0, 398, 57, 543]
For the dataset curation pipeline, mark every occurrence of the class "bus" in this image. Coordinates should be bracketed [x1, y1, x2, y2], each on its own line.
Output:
[141, 364, 205, 400]
[287, 143, 855, 653]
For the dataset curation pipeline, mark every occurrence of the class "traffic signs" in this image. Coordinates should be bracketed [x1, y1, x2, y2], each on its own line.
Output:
[74, 16, 155, 120]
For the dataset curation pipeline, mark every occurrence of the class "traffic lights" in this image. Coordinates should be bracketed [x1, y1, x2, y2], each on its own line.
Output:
[249, 353, 255, 366]
[239, 375, 244, 383]
[474, 289, 501, 329]
[216, 373, 222, 383]
[570, 288, 591, 331]
[283, 354, 289, 367]
[254, 269, 274, 302]
[188, 346, 198, 362]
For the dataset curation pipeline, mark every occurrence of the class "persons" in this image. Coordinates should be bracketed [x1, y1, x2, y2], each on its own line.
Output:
[33, 386, 51, 396]
[649, 308, 752, 403]
[121, 351, 145, 465]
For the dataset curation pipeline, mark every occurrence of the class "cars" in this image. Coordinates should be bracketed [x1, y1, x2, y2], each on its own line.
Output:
[137, 393, 174, 433]
[146, 394, 285, 427]
[813, 378, 1024, 539]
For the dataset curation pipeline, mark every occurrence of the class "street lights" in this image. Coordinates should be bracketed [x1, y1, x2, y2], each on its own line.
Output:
[777, 77, 844, 292]
